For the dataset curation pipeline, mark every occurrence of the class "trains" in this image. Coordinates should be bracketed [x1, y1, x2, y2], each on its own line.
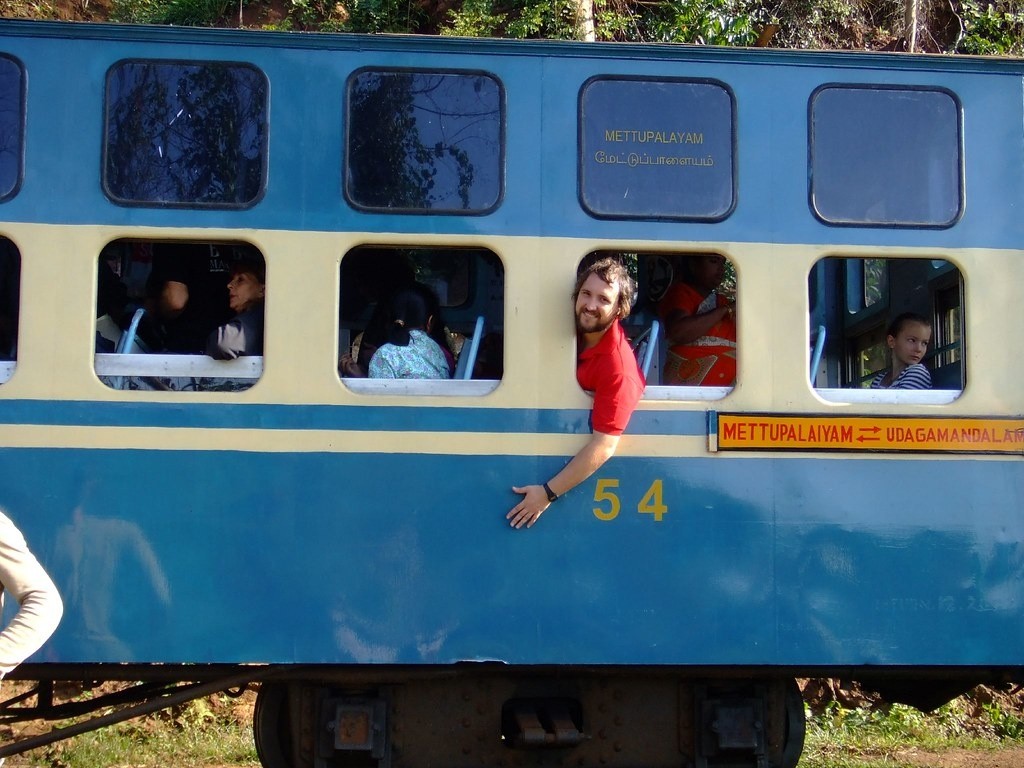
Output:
[0, 12, 1024, 768]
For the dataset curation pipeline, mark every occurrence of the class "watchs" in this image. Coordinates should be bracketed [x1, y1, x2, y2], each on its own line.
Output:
[542, 482, 559, 502]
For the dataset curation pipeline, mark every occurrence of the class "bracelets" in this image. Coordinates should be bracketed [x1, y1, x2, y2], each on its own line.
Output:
[724, 303, 733, 313]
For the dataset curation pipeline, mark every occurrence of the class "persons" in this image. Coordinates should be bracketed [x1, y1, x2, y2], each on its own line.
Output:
[0, 510, 64, 681]
[95, 244, 736, 529]
[869, 312, 935, 391]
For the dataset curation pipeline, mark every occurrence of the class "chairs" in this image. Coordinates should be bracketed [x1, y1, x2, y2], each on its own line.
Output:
[809, 325, 827, 388]
[455, 317, 487, 380]
[632, 319, 662, 386]
[109, 308, 147, 390]
